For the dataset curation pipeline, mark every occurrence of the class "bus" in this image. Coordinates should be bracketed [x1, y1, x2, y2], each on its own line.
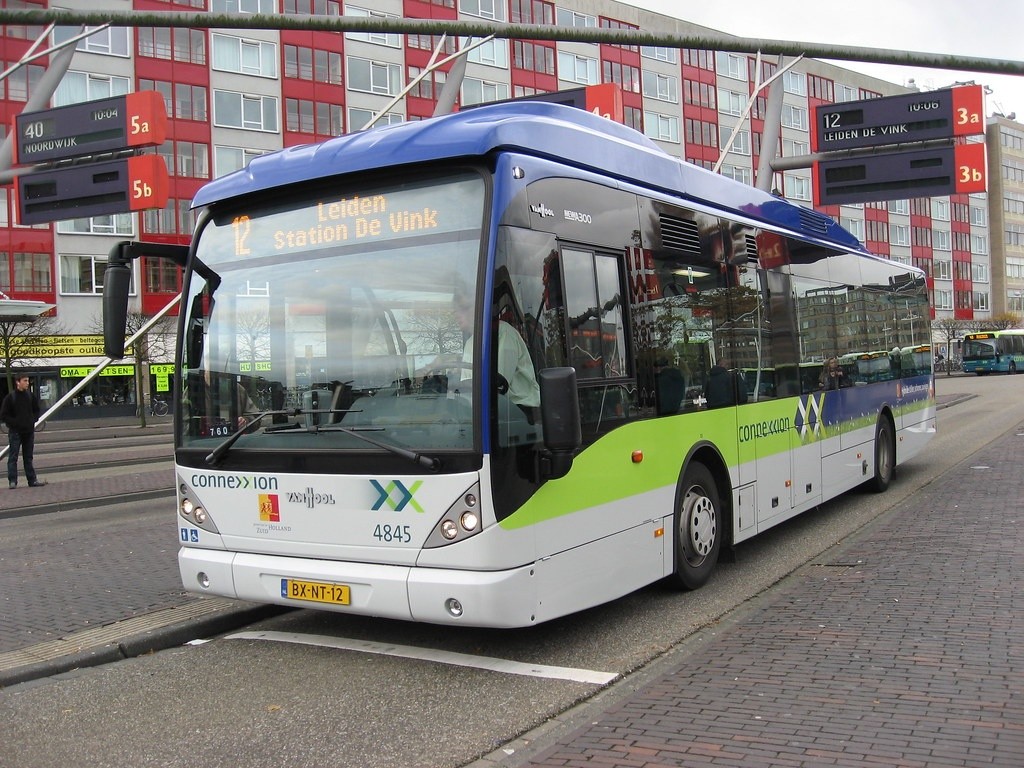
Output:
[726, 368, 777, 399]
[837, 350, 893, 386]
[899, 343, 931, 377]
[957, 327, 1024, 377]
[99, 97, 939, 632]
[797, 362, 824, 394]
[672, 336, 717, 399]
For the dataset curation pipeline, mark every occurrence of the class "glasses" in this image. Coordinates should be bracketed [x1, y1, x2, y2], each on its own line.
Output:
[453, 302, 474, 315]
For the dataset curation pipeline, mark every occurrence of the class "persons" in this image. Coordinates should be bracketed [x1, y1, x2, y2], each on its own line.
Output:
[415, 285, 540, 424]
[887, 347, 918, 377]
[655, 357, 685, 413]
[2, 373, 45, 490]
[706, 358, 747, 407]
[819, 358, 850, 390]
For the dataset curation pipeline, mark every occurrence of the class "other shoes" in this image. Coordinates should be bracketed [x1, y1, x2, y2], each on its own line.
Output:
[28, 480, 44, 486]
[9, 482, 16, 489]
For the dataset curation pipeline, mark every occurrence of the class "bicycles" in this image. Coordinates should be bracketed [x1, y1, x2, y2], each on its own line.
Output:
[0, 418, 47, 434]
[136, 393, 169, 417]
